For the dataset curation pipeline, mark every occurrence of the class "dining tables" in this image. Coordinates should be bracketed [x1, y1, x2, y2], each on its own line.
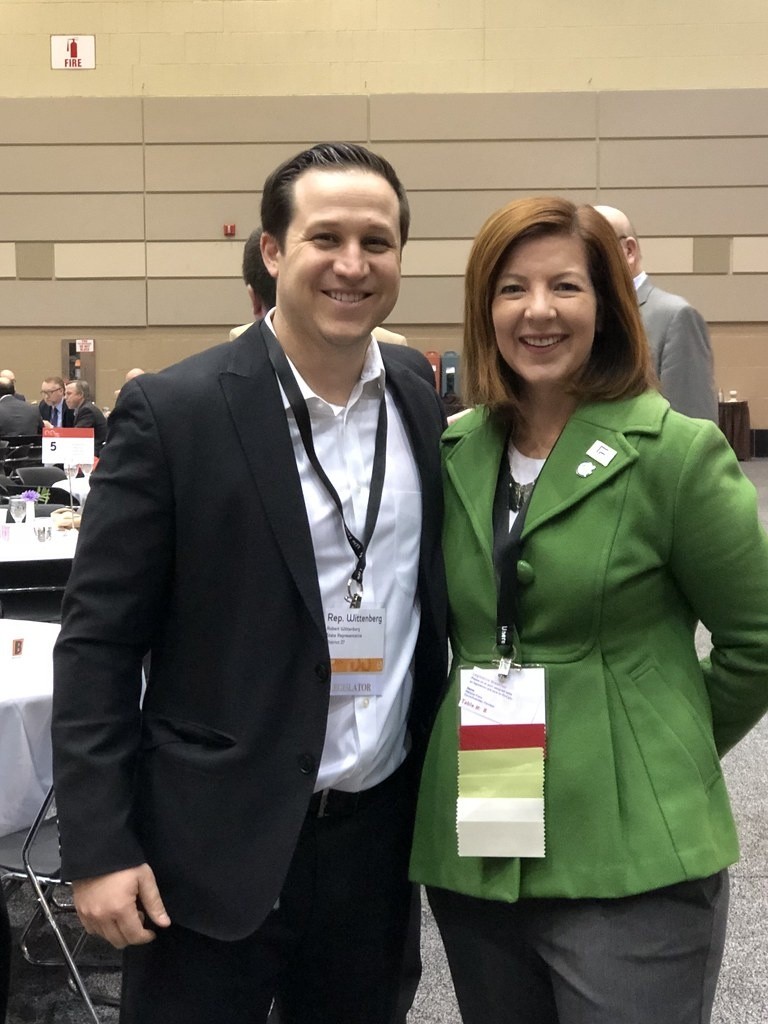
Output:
[0, 619, 146, 840]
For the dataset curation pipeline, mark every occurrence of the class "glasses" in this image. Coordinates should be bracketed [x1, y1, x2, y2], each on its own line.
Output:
[40, 386, 63, 395]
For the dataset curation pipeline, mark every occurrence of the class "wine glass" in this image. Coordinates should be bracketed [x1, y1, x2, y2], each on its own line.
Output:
[9, 496, 27, 528]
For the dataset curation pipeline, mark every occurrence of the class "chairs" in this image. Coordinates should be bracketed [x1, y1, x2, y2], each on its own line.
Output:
[0, 784, 123, 1024]
[0, 558, 74, 625]
[0, 435, 109, 523]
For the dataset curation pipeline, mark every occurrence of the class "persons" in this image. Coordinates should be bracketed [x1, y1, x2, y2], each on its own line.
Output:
[1, 138, 445, 1024]
[408, 195, 768, 1024]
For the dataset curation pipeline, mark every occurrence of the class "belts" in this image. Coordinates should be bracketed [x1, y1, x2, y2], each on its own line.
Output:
[307, 777, 414, 818]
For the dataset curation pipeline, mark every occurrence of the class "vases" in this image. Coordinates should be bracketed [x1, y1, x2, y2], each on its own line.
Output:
[26, 501, 35, 525]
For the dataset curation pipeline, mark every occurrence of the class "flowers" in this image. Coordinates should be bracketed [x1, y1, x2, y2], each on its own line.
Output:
[21, 486, 51, 505]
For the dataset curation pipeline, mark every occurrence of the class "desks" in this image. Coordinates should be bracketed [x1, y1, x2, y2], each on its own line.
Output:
[718, 400, 750, 461]
[51, 476, 92, 515]
[1, 508, 80, 561]
